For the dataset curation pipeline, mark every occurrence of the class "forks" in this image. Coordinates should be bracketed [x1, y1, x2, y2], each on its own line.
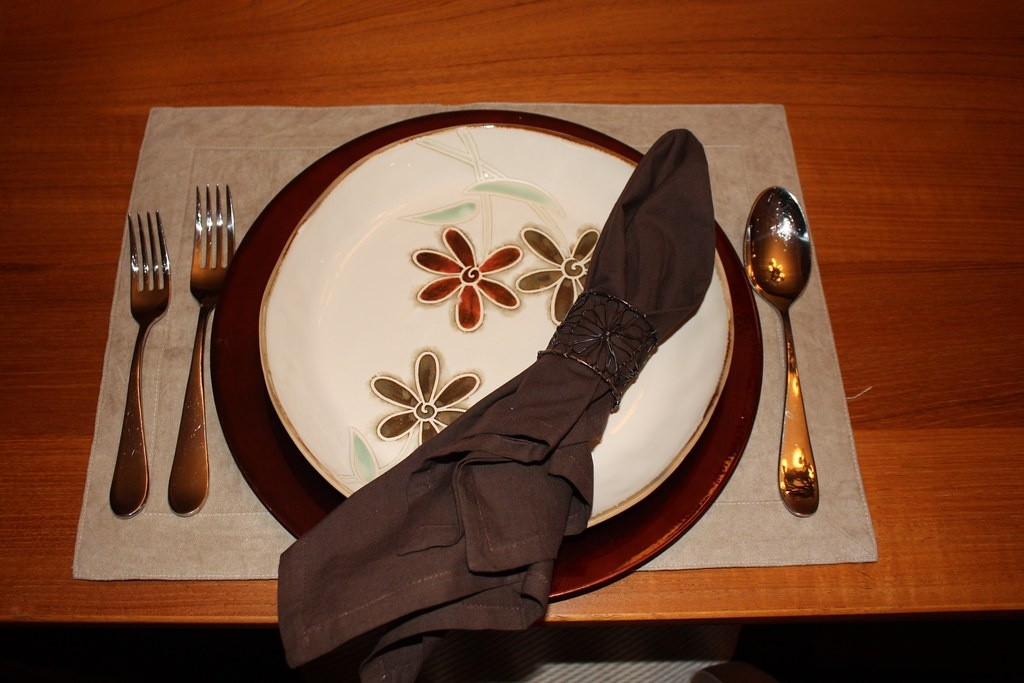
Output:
[109, 183, 236, 519]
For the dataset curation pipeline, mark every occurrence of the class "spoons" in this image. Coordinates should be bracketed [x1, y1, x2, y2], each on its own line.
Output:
[742, 185, 820, 518]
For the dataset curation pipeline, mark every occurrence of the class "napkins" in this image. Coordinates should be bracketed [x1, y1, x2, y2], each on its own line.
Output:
[277, 129, 715, 683]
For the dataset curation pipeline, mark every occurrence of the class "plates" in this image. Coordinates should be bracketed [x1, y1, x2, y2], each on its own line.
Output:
[210, 110, 766, 600]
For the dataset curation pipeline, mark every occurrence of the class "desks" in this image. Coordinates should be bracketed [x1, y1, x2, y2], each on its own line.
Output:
[0, 0, 1024, 623]
